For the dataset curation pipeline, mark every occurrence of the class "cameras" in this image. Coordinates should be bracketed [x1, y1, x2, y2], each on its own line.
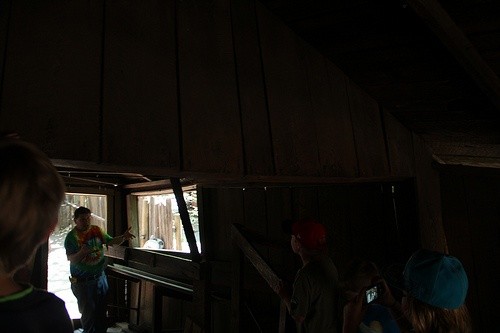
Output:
[360, 282, 385, 305]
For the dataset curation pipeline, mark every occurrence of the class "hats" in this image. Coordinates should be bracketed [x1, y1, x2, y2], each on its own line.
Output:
[403, 249, 476, 309]
[291, 220, 326, 249]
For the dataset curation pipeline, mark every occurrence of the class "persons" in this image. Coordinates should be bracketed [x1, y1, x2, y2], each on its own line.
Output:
[0, 135, 74, 333]
[65, 207, 136, 333]
[276, 216, 473, 333]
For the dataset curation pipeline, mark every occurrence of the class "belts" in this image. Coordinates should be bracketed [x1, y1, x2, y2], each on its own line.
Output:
[72, 272, 102, 281]
